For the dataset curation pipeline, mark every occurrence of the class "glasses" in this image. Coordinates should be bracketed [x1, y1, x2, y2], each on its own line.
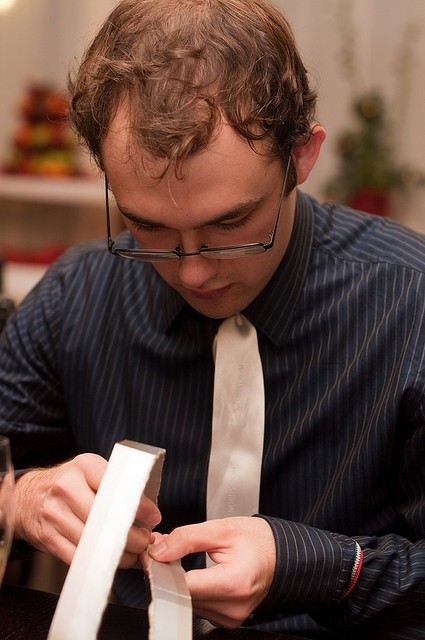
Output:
[105, 147, 294, 262]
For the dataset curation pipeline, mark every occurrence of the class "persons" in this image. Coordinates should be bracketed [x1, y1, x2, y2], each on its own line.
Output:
[0, 0, 423, 635]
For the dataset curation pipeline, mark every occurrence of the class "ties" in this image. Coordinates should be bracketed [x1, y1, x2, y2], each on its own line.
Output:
[205, 314, 265, 571]
